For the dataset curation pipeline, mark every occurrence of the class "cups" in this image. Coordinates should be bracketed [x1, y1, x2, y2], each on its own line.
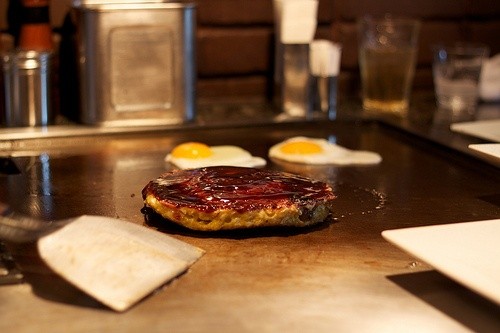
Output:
[356, 12, 422, 112]
[436, 41, 487, 116]
[8, 51, 51, 128]
